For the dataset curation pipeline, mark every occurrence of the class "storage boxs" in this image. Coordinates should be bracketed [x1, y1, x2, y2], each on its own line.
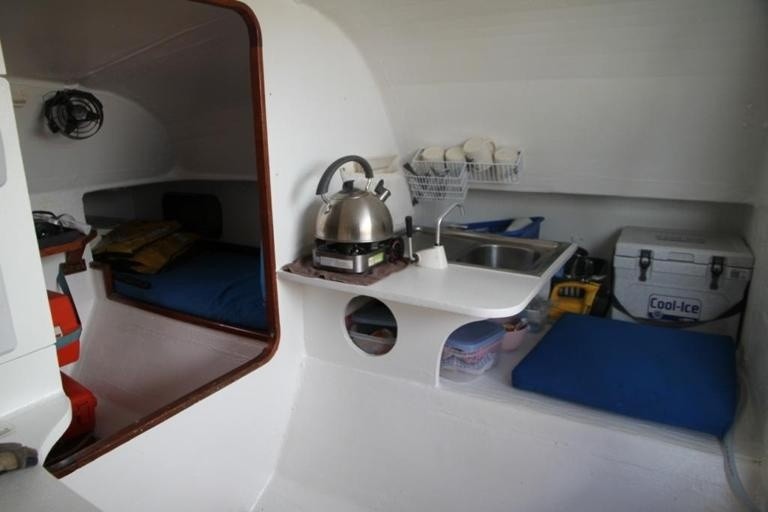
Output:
[611, 225, 754, 339]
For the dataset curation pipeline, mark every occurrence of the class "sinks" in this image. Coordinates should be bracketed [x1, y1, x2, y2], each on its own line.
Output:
[447, 234, 571, 277]
[394, 224, 482, 262]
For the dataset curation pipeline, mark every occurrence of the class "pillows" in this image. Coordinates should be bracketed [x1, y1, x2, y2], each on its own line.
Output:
[93, 216, 182, 256]
[107, 231, 197, 274]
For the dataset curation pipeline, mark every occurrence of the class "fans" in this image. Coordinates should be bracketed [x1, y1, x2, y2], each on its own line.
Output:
[42, 88, 104, 140]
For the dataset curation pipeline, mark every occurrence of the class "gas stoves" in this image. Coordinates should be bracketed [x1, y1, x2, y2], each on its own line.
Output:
[312, 238, 404, 275]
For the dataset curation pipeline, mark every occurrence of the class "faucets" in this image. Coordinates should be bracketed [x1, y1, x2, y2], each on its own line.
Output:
[434, 200, 465, 247]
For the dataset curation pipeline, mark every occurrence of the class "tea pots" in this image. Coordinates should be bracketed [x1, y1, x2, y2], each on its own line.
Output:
[314, 155, 393, 243]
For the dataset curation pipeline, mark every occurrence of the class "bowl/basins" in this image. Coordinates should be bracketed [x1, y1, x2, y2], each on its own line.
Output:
[502, 326, 529, 352]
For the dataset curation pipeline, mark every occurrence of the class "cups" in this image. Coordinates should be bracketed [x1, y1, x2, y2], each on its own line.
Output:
[421, 137, 521, 178]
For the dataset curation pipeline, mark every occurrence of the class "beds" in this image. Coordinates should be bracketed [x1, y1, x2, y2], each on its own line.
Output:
[111, 238, 268, 332]
